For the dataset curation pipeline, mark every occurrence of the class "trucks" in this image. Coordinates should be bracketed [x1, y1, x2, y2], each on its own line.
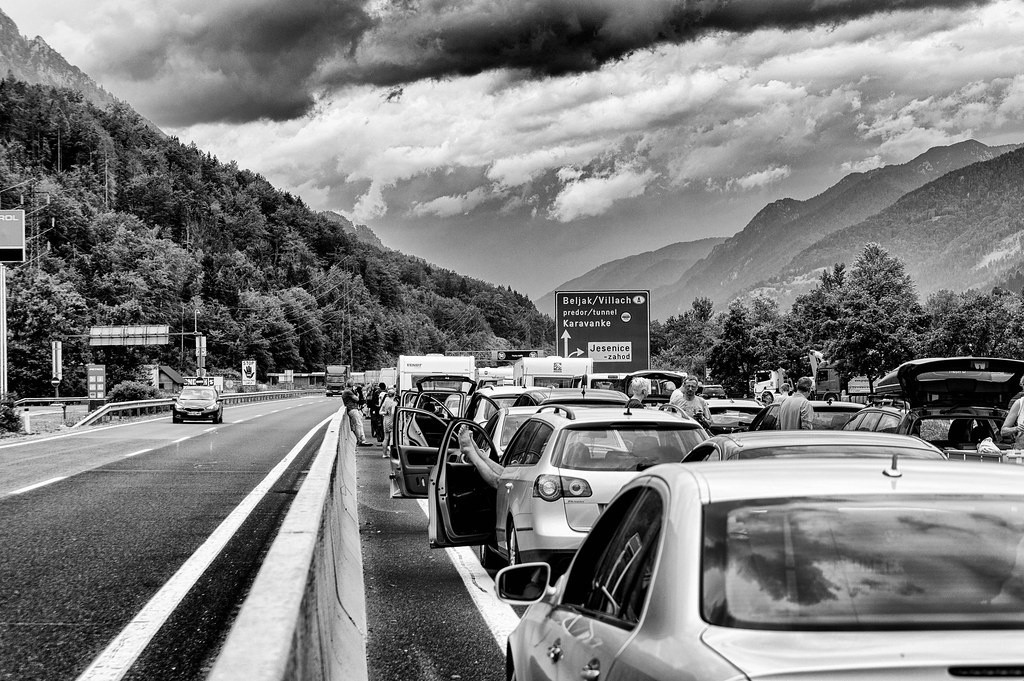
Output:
[326, 364, 351, 397]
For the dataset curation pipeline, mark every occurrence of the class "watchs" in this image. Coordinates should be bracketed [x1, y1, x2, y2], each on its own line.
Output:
[699, 416, 705, 422]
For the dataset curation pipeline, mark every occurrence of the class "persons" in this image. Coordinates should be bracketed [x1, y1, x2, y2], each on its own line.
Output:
[624, 377, 649, 409]
[778, 376, 813, 430]
[458, 424, 591, 489]
[669, 378, 687, 404]
[1000, 376, 1024, 450]
[342, 377, 397, 458]
[672, 375, 713, 430]
[778, 383, 791, 403]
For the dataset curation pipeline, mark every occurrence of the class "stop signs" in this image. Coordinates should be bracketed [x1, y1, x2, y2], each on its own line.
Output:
[196, 378, 204, 386]
[51, 377, 60, 387]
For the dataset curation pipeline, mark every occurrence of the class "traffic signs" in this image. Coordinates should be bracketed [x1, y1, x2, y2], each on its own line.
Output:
[553, 288, 652, 378]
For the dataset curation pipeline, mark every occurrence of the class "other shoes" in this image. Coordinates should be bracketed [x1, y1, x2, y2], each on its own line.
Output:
[359, 440, 373, 446]
[382, 454, 390, 458]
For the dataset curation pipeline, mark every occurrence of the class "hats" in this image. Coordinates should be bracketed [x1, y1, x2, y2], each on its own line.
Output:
[345, 378, 355, 387]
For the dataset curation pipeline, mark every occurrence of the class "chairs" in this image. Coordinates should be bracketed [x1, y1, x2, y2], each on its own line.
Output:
[831, 415, 846, 426]
[566, 442, 590, 461]
[948, 419, 974, 440]
[632, 436, 660, 457]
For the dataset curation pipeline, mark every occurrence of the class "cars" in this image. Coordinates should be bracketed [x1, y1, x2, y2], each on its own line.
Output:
[172, 386, 224, 424]
[678, 430, 949, 616]
[493, 455, 1024, 680]
[363, 353, 1024, 501]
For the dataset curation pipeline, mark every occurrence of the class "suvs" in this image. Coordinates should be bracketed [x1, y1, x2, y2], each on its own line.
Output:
[428, 402, 710, 586]
